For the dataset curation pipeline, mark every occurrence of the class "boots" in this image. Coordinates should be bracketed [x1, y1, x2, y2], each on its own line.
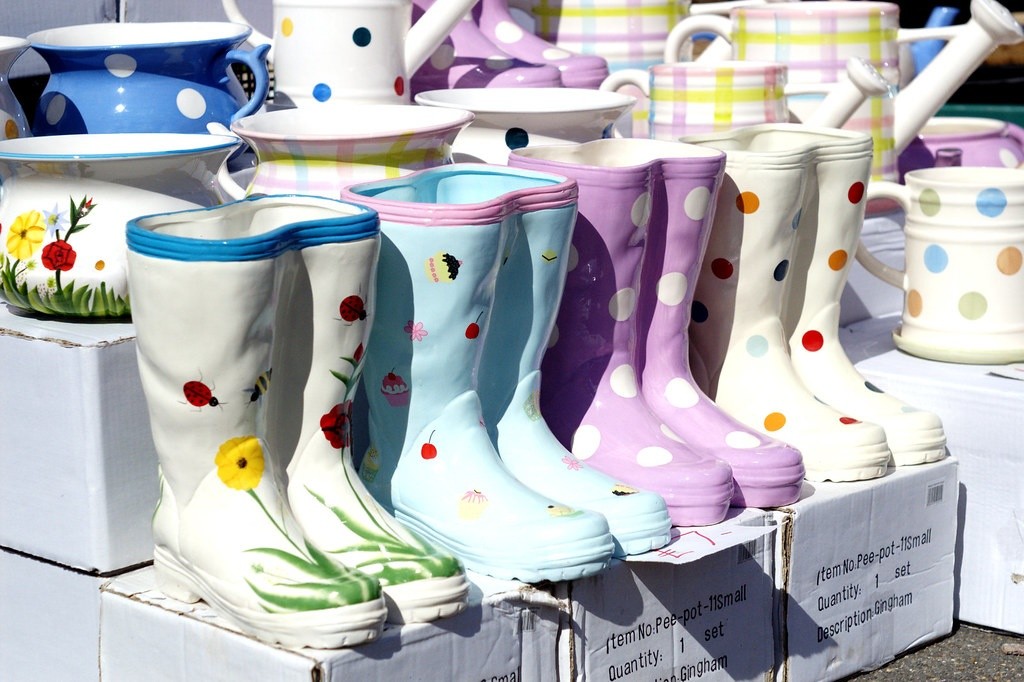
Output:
[683, 121, 949, 486]
[508, 136, 804, 532]
[126, 190, 473, 652]
[334, 158, 671, 584]
[410, 0, 608, 104]
